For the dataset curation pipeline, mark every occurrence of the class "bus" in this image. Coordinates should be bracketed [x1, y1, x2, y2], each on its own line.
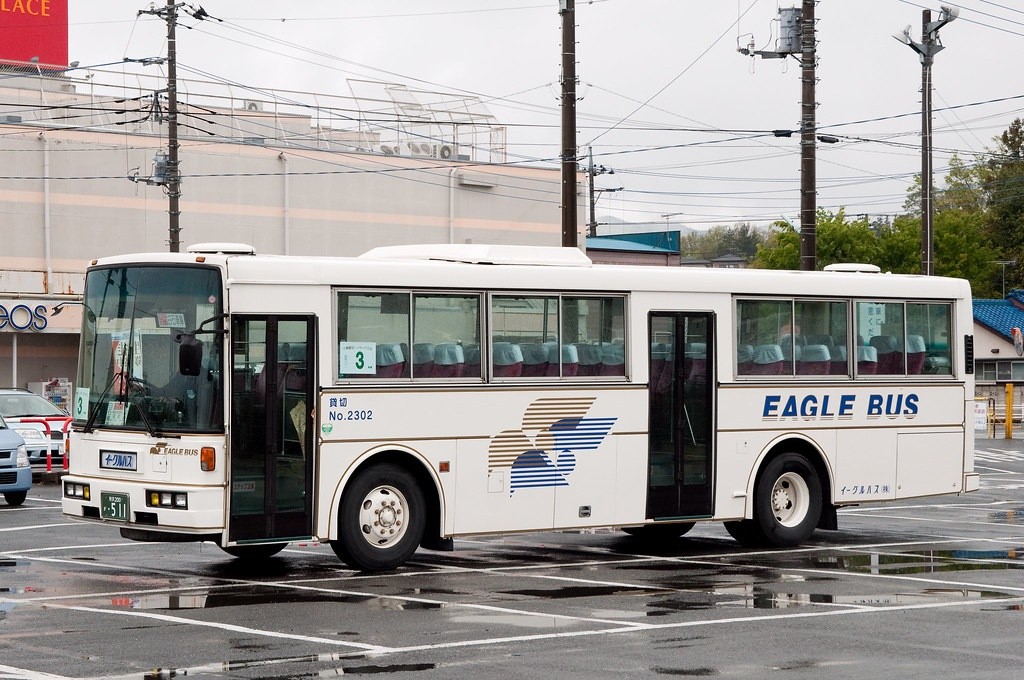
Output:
[61, 240, 981, 571]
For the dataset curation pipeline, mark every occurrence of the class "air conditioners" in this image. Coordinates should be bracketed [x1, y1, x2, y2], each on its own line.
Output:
[433, 144, 457, 159]
[243, 100, 263, 110]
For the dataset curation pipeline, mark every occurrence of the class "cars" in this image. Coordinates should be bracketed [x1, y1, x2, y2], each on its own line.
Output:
[0, 413, 32, 505]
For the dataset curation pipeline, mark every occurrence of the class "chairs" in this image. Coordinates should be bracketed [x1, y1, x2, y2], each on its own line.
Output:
[246, 335, 925, 448]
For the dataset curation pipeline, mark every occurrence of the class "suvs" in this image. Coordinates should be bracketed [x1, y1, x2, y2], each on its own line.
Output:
[0, 387, 72, 464]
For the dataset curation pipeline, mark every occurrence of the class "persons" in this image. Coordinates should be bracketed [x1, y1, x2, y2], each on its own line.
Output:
[133, 338, 216, 417]
